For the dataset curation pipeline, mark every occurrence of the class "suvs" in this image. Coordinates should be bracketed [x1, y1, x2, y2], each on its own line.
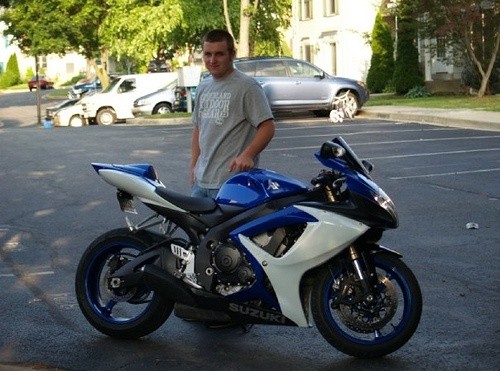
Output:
[200, 55, 369, 118]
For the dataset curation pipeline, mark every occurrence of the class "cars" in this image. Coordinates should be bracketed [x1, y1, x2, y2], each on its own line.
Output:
[24, 63, 202, 128]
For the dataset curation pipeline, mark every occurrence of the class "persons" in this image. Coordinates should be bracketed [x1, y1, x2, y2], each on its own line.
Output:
[180, 28, 276, 331]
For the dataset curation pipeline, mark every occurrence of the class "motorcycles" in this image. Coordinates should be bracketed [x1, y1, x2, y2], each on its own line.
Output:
[73, 136, 422, 359]
